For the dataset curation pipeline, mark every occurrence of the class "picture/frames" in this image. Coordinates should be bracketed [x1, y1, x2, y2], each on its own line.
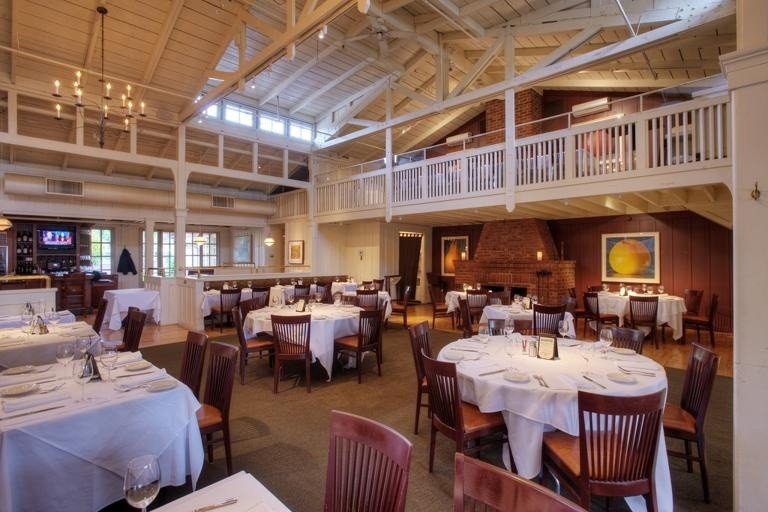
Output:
[286, 240, 305, 266]
[440, 235, 470, 278]
[600, 231, 662, 284]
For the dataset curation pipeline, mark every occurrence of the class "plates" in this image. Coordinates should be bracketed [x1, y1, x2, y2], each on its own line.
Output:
[442, 324, 660, 392]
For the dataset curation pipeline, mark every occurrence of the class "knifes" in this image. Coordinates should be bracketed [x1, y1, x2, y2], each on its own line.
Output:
[193, 498, 240, 511]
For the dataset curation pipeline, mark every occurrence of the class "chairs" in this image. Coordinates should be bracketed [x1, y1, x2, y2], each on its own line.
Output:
[270, 315, 311, 393]
[661, 344, 718, 503]
[466, 289, 508, 324]
[408, 319, 434, 436]
[324, 409, 414, 511]
[488, 317, 506, 335]
[333, 296, 388, 384]
[583, 284, 619, 341]
[604, 326, 645, 356]
[98, 306, 146, 352]
[428, 286, 455, 329]
[660, 289, 718, 346]
[512, 319, 532, 335]
[204, 278, 411, 334]
[624, 295, 659, 350]
[532, 302, 566, 336]
[538, 388, 666, 512]
[421, 348, 518, 478]
[248, 303, 366, 388]
[171, 331, 239, 477]
[454, 452, 581, 512]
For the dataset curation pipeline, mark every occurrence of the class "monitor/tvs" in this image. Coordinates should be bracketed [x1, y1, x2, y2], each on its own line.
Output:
[38, 226, 76, 250]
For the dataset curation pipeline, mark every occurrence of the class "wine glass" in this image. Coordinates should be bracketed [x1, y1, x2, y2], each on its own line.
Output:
[504, 316, 514, 339]
[462, 281, 482, 294]
[490, 294, 541, 313]
[597, 283, 668, 298]
[558, 319, 569, 345]
[0, 299, 117, 404]
[205, 276, 380, 320]
[123, 454, 163, 512]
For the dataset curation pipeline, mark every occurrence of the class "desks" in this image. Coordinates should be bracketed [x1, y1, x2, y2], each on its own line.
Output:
[339, 291, 389, 331]
[588, 292, 685, 344]
[457, 296, 479, 338]
[483, 305, 570, 337]
[104, 288, 160, 328]
[446, 291, 501, 329]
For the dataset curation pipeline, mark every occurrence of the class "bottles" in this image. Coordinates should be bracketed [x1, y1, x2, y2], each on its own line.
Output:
[32, 256, 76, 275]
[79, 255, 93, 272]
[15, 230, 32, 275]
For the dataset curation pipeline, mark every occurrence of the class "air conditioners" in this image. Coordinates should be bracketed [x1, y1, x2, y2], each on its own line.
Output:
[571, 96, 611, 119]
[446, 131, 473, 147]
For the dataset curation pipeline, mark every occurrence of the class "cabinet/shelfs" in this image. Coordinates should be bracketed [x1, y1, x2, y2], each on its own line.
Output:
[51, 274, 86, 315]
[14, 223, 91, 273]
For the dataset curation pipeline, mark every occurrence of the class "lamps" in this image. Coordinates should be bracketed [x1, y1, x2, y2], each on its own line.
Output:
[263, 233, 276, 247]
[51, 6, 146, 148]
[192, 230, 207, 246]
